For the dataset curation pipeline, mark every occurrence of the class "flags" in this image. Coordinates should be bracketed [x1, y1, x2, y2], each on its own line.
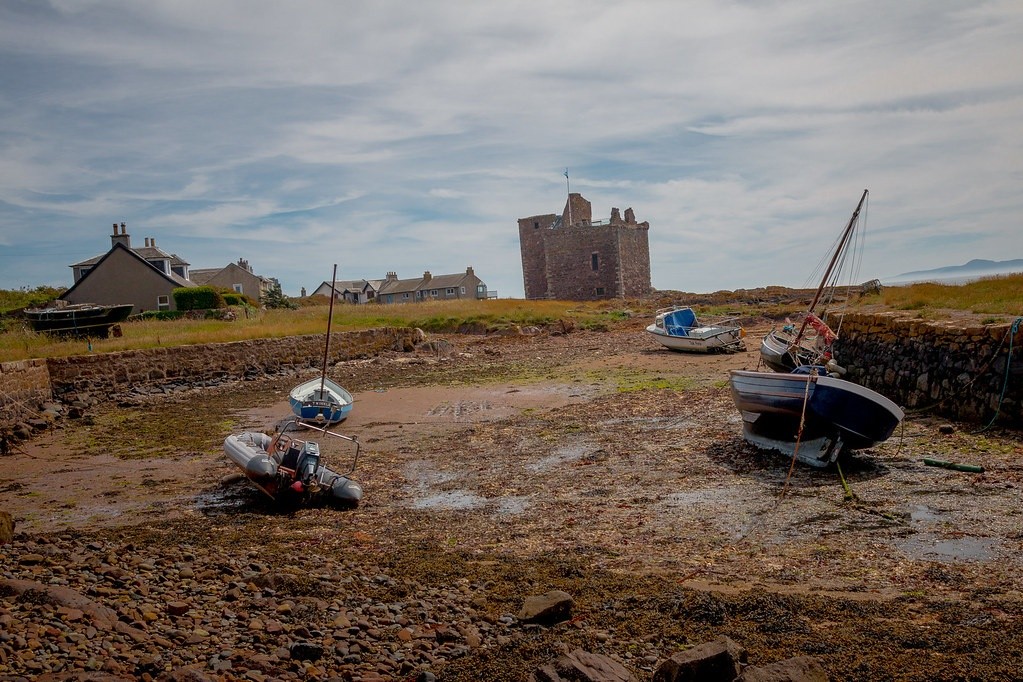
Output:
[563, 167, 569, 178]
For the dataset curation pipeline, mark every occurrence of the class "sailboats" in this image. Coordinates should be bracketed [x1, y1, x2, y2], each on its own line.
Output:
[758, 188, 870, 374]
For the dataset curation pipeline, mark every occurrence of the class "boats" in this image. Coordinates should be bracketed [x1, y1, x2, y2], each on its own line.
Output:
[289, 264, 353, 424]
[645, 305, 749, 354]
[23, 300, 134, 337]
[224, 417, 363, 515]
[728, 369, 906, 469]
